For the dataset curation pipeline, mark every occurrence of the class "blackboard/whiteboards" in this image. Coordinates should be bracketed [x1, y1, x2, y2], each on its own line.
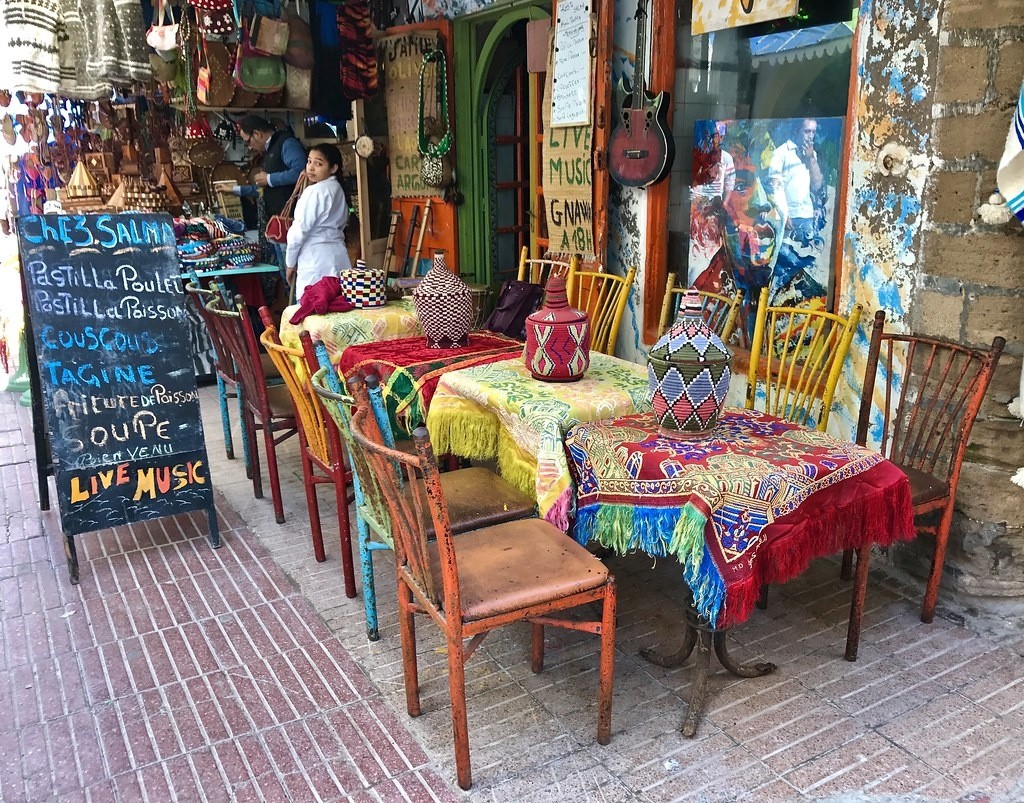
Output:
[14, 213, 215, 536]
[550, 0, 592, 127]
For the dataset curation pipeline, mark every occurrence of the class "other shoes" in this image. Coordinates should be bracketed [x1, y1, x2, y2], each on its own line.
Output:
[0, 90, 133, 215]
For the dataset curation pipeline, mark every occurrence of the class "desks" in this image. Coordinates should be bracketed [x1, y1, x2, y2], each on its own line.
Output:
[281, 298, 427, 357]
[427, 348, 667, 625]
[337, 328, 537, 472]
[567, 408, 913, 736]
[181, 262, 279, 381]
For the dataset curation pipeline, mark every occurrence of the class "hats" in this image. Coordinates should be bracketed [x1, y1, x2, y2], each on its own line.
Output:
[189, 141, 226, 168]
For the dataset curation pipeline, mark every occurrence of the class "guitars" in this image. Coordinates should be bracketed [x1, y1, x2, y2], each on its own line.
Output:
[608, 0, 675, 188]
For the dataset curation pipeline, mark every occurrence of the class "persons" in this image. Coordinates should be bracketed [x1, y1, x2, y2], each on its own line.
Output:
[285, 143, 358, 305]
[768, 118, 822, 248]
[688, 118, 736, 206]
[215, 116, 308, 314]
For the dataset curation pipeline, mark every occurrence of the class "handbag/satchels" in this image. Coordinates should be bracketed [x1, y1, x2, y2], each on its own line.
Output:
[264, 172, 311, 243]
[169, 108, 194, 182]
[186, 111, 243, 136]
[305, 112, 335, 139]
[145, 0, 290, 107]
[481, 280, 543, 337]
[283, 0, 314, 70]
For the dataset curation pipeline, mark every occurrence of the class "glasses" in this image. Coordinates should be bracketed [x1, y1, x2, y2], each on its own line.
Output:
[245, 133, 253, 147]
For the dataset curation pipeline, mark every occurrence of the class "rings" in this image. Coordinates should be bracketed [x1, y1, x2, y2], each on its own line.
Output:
[256, 182, 258, 185]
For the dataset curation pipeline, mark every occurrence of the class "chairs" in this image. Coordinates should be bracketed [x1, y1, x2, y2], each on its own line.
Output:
[560, 255, 636, 355]
[195, 292, 623, 792]
[739, 288, 862, 436]
[515, 245, 582, 320]
[753, 312, 1006, 621]
[656, 267, 742, 363]
